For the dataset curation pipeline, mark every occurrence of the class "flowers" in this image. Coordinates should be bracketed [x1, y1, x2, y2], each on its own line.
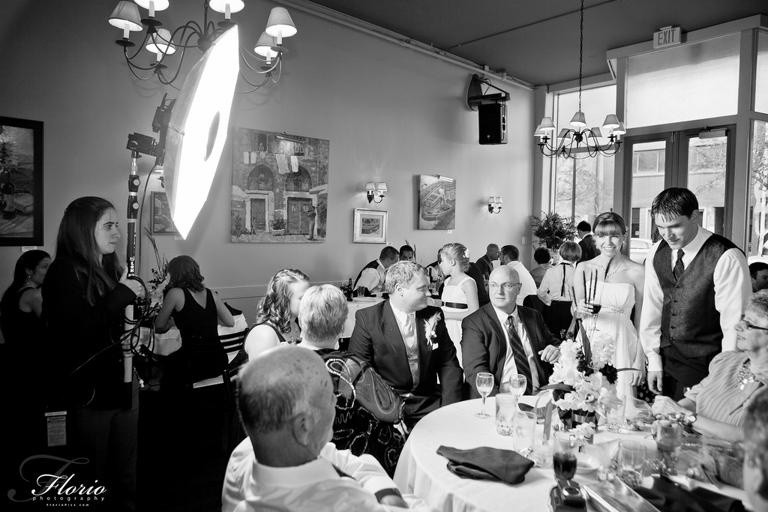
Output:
[138, 227, 171, 309]
[534, 320, 642, 440]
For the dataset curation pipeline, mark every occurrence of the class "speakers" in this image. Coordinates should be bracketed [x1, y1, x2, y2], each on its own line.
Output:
[479, 103, 508, 145]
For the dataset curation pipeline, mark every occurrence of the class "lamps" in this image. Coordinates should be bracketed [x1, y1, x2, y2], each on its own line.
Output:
[364, 181, 388, 205]
[106, 0, 300, 97]
[533, 0, 625, 160]
[487, 196, 504, 215]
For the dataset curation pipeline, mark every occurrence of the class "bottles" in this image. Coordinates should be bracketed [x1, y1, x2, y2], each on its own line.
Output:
[347, 278, 353, 301]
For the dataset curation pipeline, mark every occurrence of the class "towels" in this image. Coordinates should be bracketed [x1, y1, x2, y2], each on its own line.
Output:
[437, 443, 537, 486]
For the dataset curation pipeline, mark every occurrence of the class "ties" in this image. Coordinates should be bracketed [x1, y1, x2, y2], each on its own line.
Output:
[505, 317, 533, 394]
[402, 315, 418, 390]
[673, 250, 685, 281]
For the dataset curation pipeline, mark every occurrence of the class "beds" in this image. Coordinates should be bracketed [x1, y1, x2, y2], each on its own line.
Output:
[393, 394, 651, 512]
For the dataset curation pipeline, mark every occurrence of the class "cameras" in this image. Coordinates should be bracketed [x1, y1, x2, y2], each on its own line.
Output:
[557, 478, 585, 506]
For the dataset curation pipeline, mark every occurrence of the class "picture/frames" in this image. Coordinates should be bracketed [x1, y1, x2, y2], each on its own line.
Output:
[352, 208, 388, 245]
[149, 191, 180, 236]
[0, 116, 45, 246]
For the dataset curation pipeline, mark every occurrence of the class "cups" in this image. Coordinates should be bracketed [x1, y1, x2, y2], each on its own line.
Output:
[357, 285, 364, 298]
[657, 420, 683, 459]
[496, 373, 647, 480]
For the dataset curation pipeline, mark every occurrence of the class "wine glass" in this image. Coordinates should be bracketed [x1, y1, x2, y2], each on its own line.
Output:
[588, 295, 601, 333]
[474, 372, 494, 418]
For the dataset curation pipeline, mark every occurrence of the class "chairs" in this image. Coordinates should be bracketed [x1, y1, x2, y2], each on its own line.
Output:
[205, 332, 247, 451]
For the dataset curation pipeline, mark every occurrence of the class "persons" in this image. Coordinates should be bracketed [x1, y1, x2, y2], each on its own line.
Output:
[38, 195, 149, 511]
[0, 248, 53, 345]
[152, 253, 236, 385]
[215, 185, 767, 512]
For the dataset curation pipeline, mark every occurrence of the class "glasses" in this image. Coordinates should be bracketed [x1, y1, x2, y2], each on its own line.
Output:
[490, 281, 520, 289]
[739, 314, 768, 330]
[733, 441, 747, 463]
